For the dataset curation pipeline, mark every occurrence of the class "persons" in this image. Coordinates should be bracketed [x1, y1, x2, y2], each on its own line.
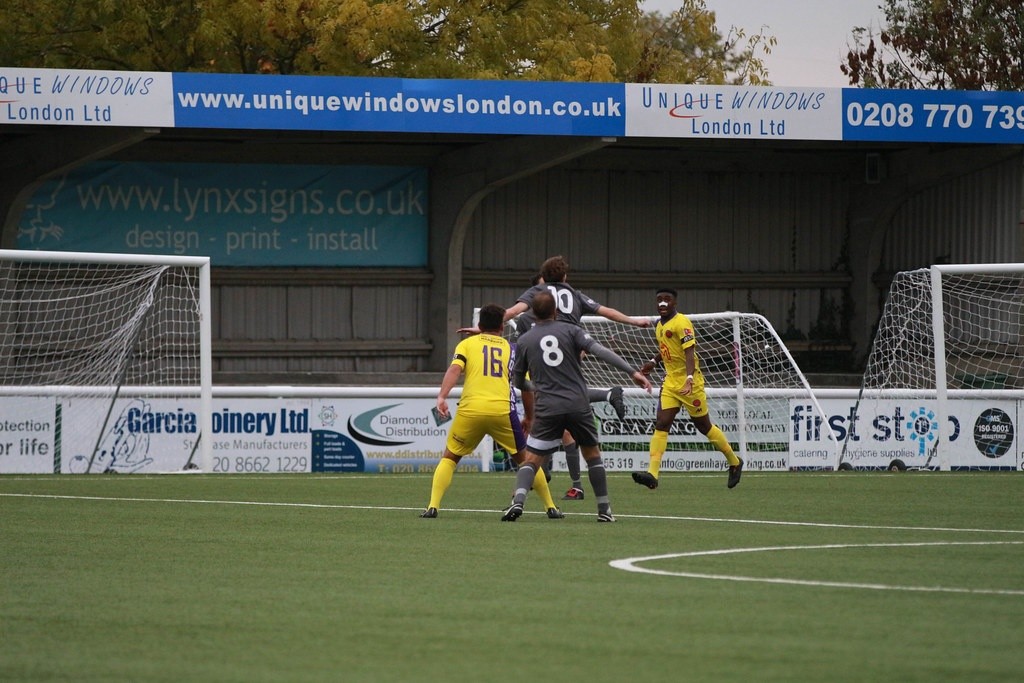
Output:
[453, 255, 652, 501]
[632, 287, 744, 490]
[419, 303, 566, 519]
[500, 291, 653, 522]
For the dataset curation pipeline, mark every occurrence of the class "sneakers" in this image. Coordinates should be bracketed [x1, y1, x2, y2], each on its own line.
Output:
[500, 503, 523, 523]
[597, 511, 618, 523]
[725, 456, 744, 488]
[547, 505, 566, 519]
[609, 384, 626, 422]
[631, 470, 658, 489]
[562, 487, 584, 501]
[420, 507, 438, 519]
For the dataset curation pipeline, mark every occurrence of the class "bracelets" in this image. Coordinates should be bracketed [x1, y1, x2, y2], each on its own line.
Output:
[687, 375, 693, 380]
[651, 358, 657, 365]
